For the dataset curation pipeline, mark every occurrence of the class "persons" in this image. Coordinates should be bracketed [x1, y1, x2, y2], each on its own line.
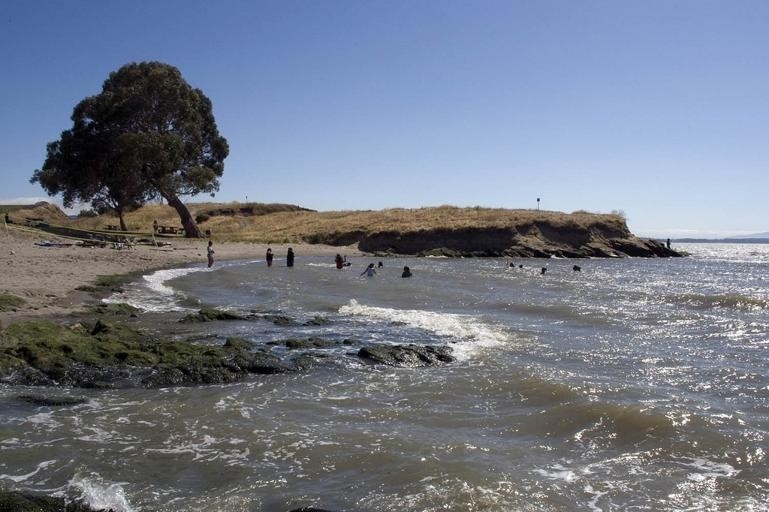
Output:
[667, 239, 670, 248]
[335, 254, 351, 269]
[541, 267, 547, 274]
[402, 266, 412, 278]
[287, 248, 294, 267]
[266, 248, 274, 267]
[207, 241, 215, 267]
[361, 261, 383, 277]
[153, 220, 159, 234]
